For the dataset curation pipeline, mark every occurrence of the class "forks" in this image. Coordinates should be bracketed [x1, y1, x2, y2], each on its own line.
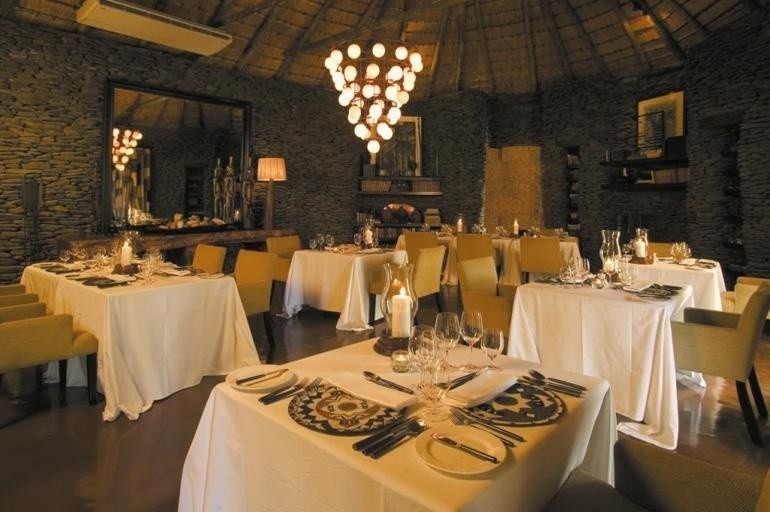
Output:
[259, 376, 327, 404]
[517, 368, 587, 400]
[446, 407, 523, 447]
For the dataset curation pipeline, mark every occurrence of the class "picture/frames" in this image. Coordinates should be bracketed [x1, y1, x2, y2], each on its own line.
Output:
[365, 114, 421, 180]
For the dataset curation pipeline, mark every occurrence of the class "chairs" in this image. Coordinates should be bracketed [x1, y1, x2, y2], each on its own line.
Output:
[454, 255, 517, 355]
[395, 221, 727, 309]
[192, 243, 276, 351]
[1, 282, 99, 410]
[546, 437, 768, 512]
[668, 280, 770, 449]
[266, 235, 303, 303]
[367, 245, 448, 328]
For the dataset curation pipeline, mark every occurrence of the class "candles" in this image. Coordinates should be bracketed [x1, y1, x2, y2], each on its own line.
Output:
[363, 228, 375, 245]
[388, 287, 415, 337]
[116, 240, 135, 270]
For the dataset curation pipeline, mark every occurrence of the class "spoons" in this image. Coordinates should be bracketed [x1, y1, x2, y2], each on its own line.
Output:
[362, 370, 417, 396]
[355, 412, 425, 458]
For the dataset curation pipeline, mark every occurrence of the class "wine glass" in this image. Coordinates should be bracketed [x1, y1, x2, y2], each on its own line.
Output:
[308, 213, 379, 254]
[58, 231, 161, 286]
[558, 254, 632, 289]
[411, 310, 504, 419]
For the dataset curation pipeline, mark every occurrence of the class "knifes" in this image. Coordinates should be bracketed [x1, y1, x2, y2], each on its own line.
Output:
[235, 366, 287, 386]
[433, 431, 500, 463]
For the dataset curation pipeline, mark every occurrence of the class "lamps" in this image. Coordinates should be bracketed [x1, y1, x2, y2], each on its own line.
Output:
[257, 154, 287, 231]
[326, 19, 423, 166]
[111, 115, 144, 174]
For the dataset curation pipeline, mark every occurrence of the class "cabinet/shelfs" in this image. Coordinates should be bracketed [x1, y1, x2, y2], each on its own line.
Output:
[717, 144, 749, 278]
[602, 154, 688, 194]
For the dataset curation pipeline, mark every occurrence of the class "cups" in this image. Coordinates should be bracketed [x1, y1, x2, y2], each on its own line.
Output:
[599, 229, 622, 267]
[378, 261, 419, 335]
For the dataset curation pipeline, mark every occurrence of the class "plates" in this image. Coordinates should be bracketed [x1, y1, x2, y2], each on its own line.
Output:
[415, 423, 509, 475]
[224, 364, 294, 391]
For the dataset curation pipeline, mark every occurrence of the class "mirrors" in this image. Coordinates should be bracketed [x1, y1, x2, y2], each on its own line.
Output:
[99, 74, 254, 233]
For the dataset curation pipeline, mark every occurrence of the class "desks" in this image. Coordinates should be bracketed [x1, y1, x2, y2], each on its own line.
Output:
[276, 244, 412, 333]
[175, 328, 621, 511]
[510, 273, 694, 450]
[18, 257, 260, 424]
